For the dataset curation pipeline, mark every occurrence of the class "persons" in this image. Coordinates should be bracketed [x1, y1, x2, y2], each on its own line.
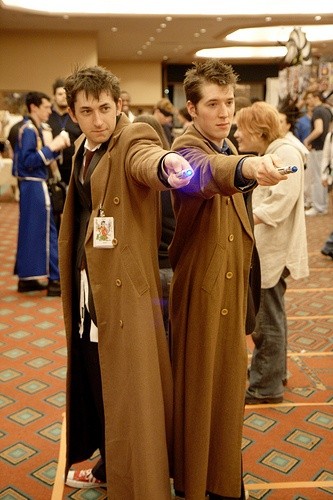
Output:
[51, 66, 195, 500]
[227, 90, 333, 403]
[169, 59, 287, 500]
[121, 91, 192, 144]
[8, 81, 83, 297]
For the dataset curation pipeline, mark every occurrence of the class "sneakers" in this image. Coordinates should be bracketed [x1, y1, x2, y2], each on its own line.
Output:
[65, 469, 108, 489]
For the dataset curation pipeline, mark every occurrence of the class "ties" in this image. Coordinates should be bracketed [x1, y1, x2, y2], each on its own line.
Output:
[81, 149, 96, 270]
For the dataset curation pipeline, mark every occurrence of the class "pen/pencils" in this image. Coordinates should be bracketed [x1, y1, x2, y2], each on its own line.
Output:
[276, 166, 297, 175]
[177, 170, 192, 179]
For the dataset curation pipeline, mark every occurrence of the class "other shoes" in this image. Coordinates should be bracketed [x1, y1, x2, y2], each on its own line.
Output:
[46, 278, 61, 296]
[320, 247, 333, 259]
[246, 368, 287, 386]
[245, 388, 284, 405]
[17, 278, 45, 292]
[305, 207, 327, 216]
